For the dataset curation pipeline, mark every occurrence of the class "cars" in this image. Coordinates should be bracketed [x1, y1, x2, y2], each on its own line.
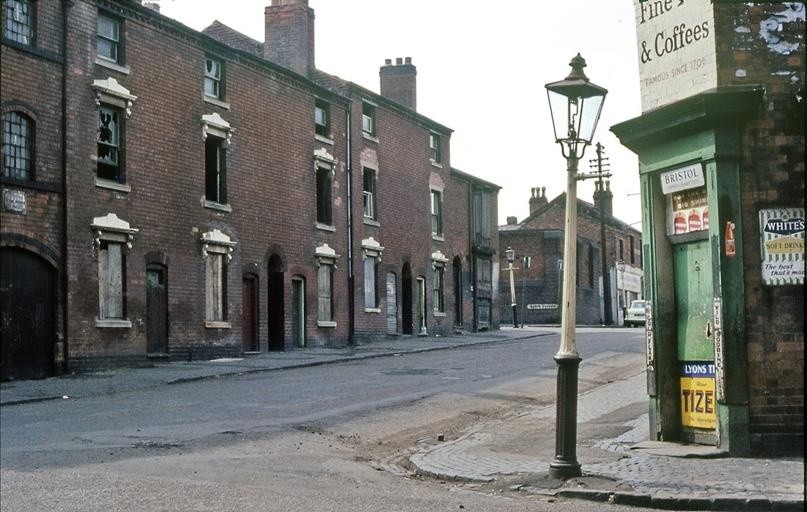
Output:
[626, 300, 645, 328]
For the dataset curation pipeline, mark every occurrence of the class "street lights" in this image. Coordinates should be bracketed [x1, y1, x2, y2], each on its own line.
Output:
[544, 51, 608, 479]
[500, 246, 521, 328]
